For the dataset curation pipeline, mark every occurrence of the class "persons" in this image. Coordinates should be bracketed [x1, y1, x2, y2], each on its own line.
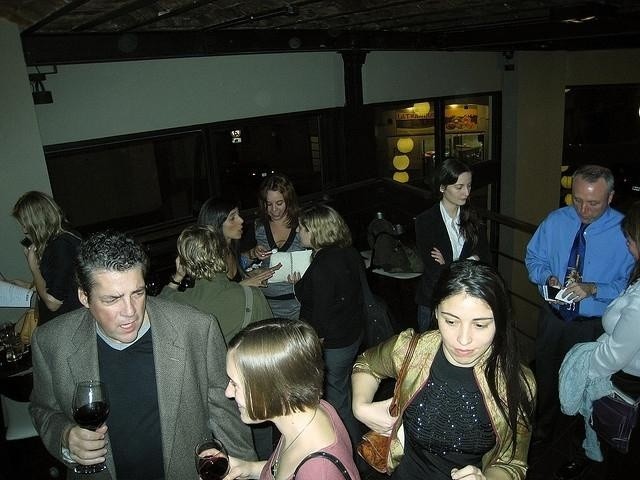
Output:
[156, 223, 276, 347]
[29, 227, 261, 480]
[195, 318, 361, 480]
[582, 198, 640, 479]
[293, 203, 370, 445]
[242, 171, 310, 322]
[11, 190, 86, 323]
[414, 156, 486, 311]
[525, 163, 636, 480]
[197, 194, 282, 287]
[349, 258, 540, 480]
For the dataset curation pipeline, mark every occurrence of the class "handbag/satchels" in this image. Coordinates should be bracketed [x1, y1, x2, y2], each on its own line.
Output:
[362, 293, 395, 347]
[588, 397, 637, 454]
[355, 401, 402, 474]
[13, 308, 41, 346]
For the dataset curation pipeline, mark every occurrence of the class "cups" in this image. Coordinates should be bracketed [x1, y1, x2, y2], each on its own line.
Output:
[195, 439, 229, 480]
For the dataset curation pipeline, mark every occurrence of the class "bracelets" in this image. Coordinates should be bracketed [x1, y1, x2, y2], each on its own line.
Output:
[168, 275, 183, 287]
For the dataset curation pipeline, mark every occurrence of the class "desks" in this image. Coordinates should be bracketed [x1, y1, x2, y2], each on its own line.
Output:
[365, 260, 425, 282]
[0, 349, 33, 378]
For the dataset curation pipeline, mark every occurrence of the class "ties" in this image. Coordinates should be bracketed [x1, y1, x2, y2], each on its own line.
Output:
[558, 222, 590, 324]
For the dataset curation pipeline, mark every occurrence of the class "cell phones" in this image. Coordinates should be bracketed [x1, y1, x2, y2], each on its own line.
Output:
[547, 286, 560, 299]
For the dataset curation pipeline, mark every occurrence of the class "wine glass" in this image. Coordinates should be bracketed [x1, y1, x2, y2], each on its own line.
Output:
[71, 379, 108, 473]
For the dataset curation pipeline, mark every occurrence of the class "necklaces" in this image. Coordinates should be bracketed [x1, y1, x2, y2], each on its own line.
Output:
[272, 406, 318, 477]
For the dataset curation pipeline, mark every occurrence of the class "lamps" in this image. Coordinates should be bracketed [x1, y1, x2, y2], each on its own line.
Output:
[392, 137, 414, 183]
[413, 101, 431, 117]
